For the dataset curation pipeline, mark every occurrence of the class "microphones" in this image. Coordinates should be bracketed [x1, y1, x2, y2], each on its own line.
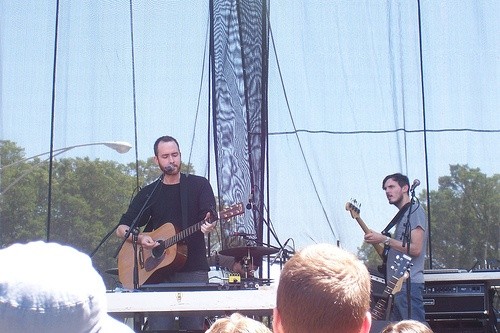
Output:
[410, 179, 420, 191]
[246, 186, 254, 209]
[229, 231, 254, 236]
[271, 239, 289, 265]
[164, 164, 175, 173]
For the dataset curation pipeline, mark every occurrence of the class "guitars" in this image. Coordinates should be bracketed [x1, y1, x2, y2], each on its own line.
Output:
[370, 254, 414, 320]
[345, 197, 386, 262]
[117, 202, 245, 290]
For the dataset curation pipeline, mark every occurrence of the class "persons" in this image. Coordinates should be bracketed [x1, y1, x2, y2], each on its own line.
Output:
[205, 313, 272, 333]
[382, 319, 432, 333]
[0, 241, 135, 333]
[364, 173, 427, 321]
[273, 244, 371, 333]
[209, 254, 261, 277]
[117, 136, 217, 330]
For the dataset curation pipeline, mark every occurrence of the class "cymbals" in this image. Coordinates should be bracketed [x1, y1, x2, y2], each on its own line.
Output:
[218, 247, 280, 258]
[102, 268, 119, 275]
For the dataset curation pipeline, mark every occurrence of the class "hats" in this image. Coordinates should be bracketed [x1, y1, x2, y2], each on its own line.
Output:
[0, 241, 136, 333]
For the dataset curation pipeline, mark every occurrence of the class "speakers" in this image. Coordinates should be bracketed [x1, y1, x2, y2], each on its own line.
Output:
[426, 318, 492, 333]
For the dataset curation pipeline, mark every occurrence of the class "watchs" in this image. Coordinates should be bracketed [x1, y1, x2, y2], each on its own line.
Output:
[384, 237, 390, 245]
